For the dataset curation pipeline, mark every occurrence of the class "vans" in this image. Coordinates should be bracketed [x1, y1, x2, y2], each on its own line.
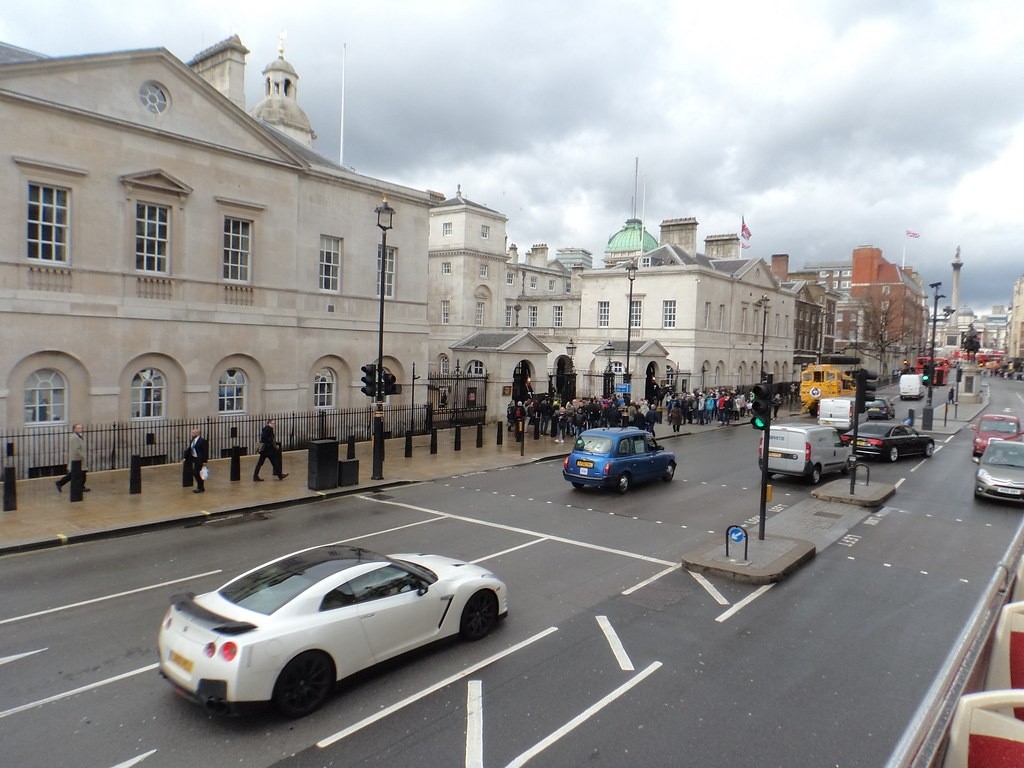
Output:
[899, 374, 925, 400]
[758, 423, 853, 487]
[817, 395, 855, 433]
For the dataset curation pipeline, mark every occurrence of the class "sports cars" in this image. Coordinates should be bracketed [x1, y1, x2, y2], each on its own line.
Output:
[157, 543, 510, 722]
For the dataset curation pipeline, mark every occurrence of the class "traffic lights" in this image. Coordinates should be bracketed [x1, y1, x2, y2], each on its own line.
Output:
[923, 365, 931, 387]
[360, 363, 376, 397]
[855, 368, 878, 414]
[384, 372, 396, 395]
[750, 382, 774, 431]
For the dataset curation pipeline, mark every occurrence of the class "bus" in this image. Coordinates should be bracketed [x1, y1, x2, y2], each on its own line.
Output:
[916, 347, 1024, 386]
[799, 363, 857, 417]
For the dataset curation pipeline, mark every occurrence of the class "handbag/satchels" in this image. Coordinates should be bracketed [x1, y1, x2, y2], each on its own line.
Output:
[255, 442, 264, 454]
[199, 466, 210, 480]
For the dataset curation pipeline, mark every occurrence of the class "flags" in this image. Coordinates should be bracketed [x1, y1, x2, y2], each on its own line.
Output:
[907, 231, 919, 238]
[738, 241, 750, 249]
[741, 219, 751, 240]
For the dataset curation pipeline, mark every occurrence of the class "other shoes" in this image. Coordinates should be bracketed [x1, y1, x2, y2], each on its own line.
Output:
[83, 487, 90, 492]
[279, 473, 289, 479]
[56, 481, 62, 492]
[253, 478, 264, 481]
[193, 489, 205, 493]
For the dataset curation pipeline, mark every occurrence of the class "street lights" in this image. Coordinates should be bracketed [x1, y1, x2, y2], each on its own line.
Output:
[926, 282, 943, 407]
[760, 294, 771, 383]
[621, 261, 640, 428]
[370, 196, 396, 481]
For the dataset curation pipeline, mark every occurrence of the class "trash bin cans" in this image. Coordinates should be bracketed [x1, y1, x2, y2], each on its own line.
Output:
[308, 439, 338, 491]
[656, 408, 662, 424]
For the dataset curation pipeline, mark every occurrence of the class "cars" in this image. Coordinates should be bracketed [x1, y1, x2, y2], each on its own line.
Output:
[972, 439, 1024, 504]
[563, 426, 677, 496]
[840, 420, 936, 464]
[865, 396, 895, 420]
[969, 414, 1024, 457]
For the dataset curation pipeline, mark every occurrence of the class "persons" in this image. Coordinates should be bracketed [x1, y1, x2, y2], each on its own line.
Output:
[189, 429, 208, 493]
[252, 420, 290, 481]
[666, 387, 782, 432]
[55, 424, 90, 492]
[965, 323, 977, 339]
[507, 394, 660, 443]
[948, 386, 954, 404]
[787, 382, 800, 401]
[652, 378, 669, 406]
